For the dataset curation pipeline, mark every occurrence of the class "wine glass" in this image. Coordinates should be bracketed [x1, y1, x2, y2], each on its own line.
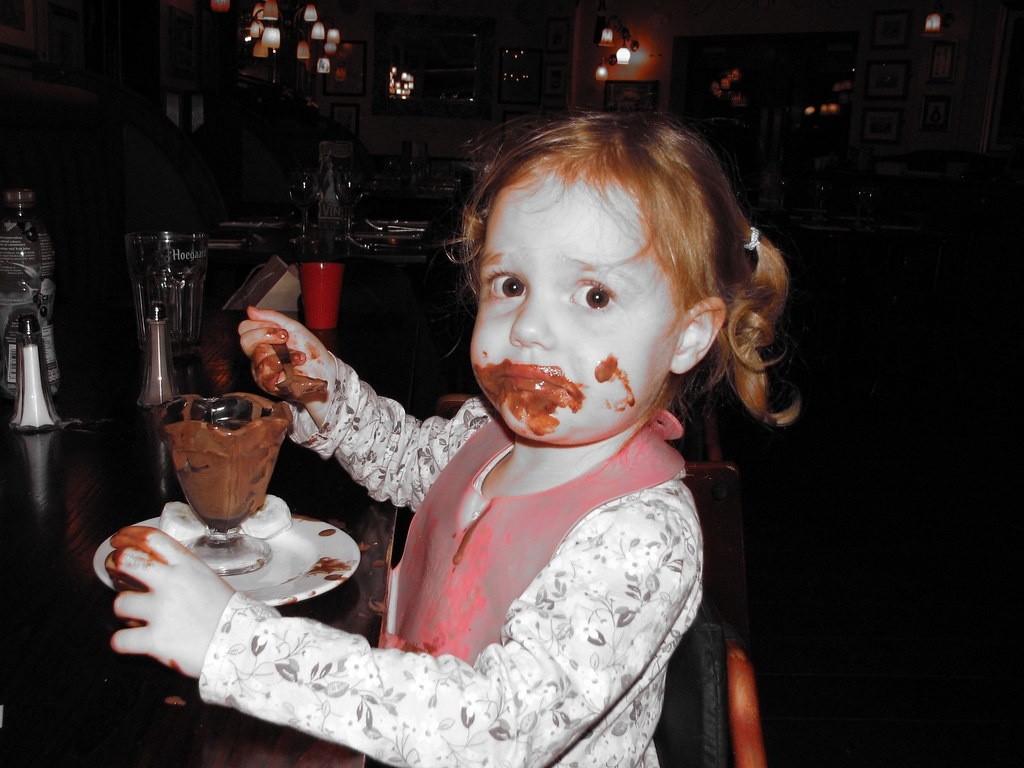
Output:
[336, 169, 367, 243]
[160, 392, 293, 573]
[287, 172, 319, 249]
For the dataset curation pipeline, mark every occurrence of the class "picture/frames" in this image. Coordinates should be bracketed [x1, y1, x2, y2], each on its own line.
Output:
[322, 40, 367, 96]
[861, 9, 958, 145]
[604, 79, 660, 112]
[978, 1, 1024, 158]
[330, 103, 360, 137]
[498, 15, 575, 131]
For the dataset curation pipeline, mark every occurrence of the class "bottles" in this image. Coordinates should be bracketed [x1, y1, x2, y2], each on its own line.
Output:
[0, 189, 61, 402]
[137, 301, 181, 406]
[8, 316, 64, 432]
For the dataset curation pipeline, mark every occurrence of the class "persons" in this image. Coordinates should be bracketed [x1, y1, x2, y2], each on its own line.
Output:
[105, 110, 802, 768]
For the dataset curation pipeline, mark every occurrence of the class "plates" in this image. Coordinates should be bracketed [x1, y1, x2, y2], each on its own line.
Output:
[93, 516, 362, 608]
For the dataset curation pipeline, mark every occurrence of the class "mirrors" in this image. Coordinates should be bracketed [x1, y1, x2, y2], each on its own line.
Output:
[374, 10, 493, 121]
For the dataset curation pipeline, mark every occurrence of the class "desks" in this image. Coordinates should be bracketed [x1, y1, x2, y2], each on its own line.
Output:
[755, 207, 928, 322]
[1, 155, 476, 768]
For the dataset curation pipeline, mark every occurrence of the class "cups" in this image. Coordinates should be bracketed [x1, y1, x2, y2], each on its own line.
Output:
[300, 260, 344, 332]
[123, 233, 207, 346]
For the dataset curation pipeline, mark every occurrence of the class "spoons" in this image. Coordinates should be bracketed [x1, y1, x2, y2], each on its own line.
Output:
[271, 339, 328, 396]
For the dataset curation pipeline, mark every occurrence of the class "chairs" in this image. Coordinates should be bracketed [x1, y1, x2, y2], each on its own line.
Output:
[656, 462, 766, 766]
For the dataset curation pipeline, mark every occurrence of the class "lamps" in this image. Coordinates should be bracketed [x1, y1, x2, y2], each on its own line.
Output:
[595, 13, 641, 80]
[210, 0, 341, 74]
[923, 1, 955, 36]
